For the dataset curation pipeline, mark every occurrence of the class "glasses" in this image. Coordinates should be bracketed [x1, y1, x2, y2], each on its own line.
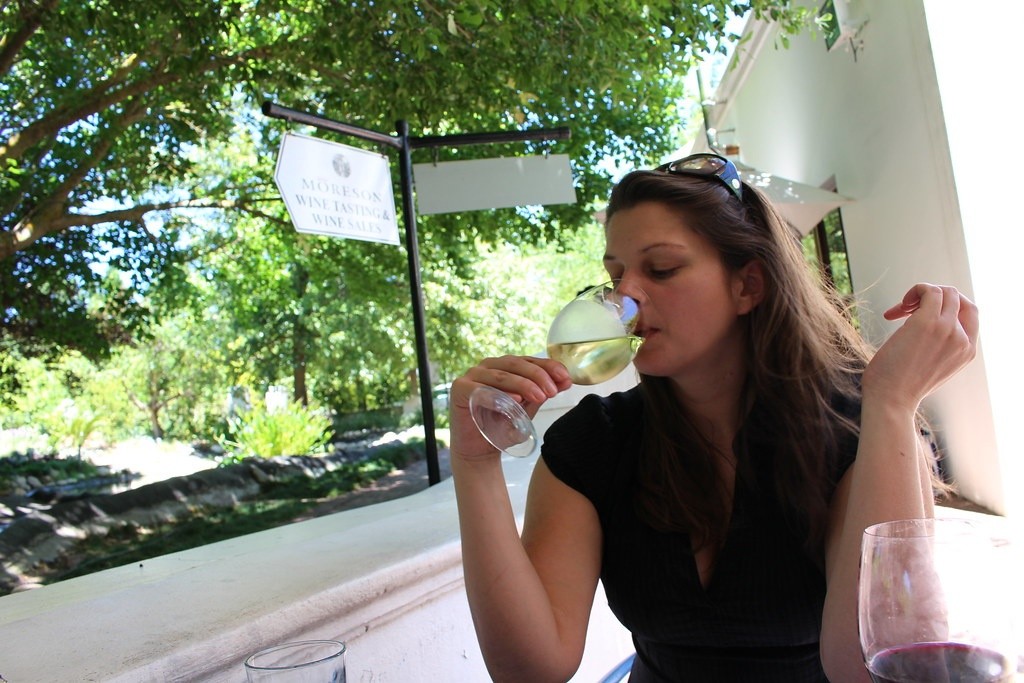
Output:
[653, 153, 742, 202]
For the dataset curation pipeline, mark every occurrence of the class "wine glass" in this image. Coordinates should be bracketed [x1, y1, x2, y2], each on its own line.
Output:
[469, 279, 659, 458]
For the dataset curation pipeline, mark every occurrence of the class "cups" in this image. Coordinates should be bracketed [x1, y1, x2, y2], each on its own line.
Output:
[244, 639, 347, 683]
[857, 518, 1024, 683]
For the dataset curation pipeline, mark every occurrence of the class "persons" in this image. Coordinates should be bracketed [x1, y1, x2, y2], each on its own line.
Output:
[449, 170, 980, 683]
